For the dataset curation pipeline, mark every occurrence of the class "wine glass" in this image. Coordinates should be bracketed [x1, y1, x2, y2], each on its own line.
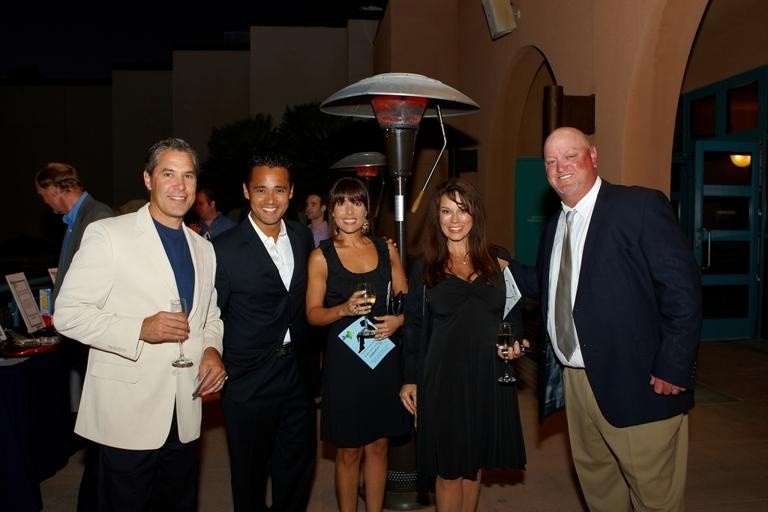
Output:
[170, 298, 193, 368]
[354, 284, 376, 338]
[497, 321, 517, 382]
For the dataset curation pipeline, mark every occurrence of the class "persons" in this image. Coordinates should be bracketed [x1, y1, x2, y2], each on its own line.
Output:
[305, 177, 412, 510]
[209, 156, 398, 511]
[190, 188, 237, 239]
[36, 163, 147, 304]
[305, 194, 331, 247]
[537, 127, 698, 510]
[400, 178, 529, 510]
[54, 138, 226, 512]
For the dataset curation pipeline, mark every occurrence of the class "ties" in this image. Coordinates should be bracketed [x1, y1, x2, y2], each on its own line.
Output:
[556, 211, 577, 362]
